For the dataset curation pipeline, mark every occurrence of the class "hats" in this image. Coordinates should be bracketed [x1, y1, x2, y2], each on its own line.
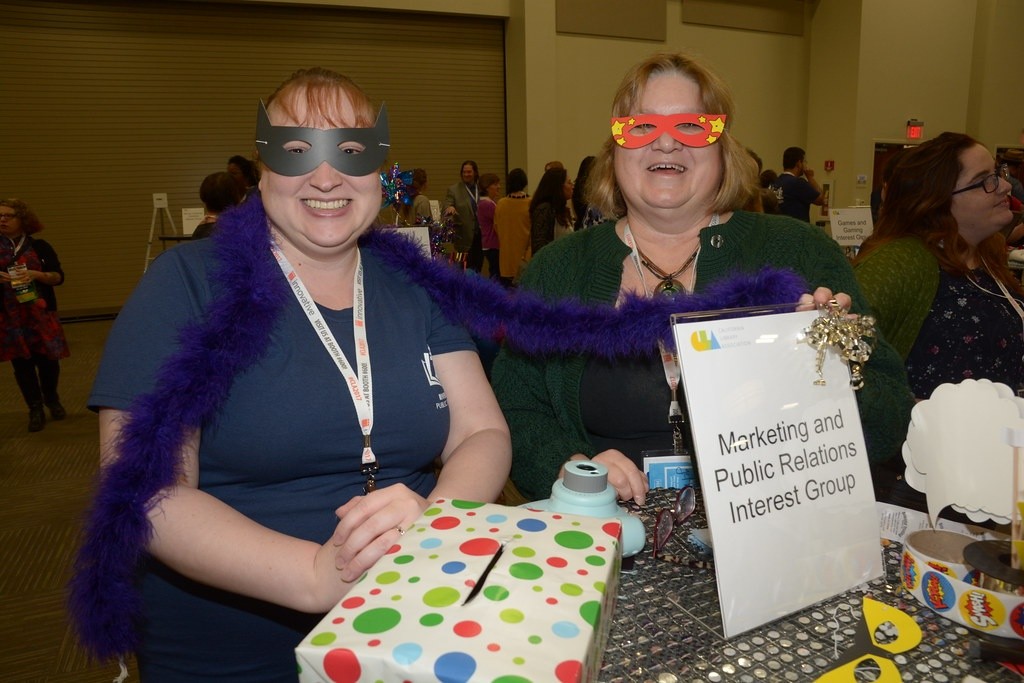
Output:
[1000, 149, 1024, 162]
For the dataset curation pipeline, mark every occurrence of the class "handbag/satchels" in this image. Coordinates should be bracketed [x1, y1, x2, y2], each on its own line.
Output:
[512, 254, 530, 284]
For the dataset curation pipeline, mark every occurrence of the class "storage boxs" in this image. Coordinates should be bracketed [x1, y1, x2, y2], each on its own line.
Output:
[293, 497, 622, 683]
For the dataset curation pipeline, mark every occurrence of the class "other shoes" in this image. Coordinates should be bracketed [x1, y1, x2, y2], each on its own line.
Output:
[45, 400, 66, 419]
[28, 408, 45, 431]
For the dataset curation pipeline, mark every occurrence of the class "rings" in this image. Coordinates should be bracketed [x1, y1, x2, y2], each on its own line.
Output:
[396, 527, 404, 535]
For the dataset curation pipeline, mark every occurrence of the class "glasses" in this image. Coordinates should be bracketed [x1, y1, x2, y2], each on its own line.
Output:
[952, 163, 1009, 194]
[0, 213, 18, 221]
[653, 486, 714, 569]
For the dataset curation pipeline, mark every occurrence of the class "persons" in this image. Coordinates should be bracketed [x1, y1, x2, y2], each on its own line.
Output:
[399, 156, 606, 286]
[88, 67, 512, 683]
[490, 54, 913, 503]
[0, 199, 72, 431]
[750, 146, 824, 223]
[998, 147, 1024, 246]
[192, 156, 256, 241]
[851, 130, 1024, 529]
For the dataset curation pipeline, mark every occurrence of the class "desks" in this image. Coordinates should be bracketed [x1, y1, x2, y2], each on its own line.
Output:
[600, 483, 1024, 683]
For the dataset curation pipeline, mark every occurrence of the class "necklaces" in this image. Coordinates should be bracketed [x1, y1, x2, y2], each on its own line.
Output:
[638, 244, 700, 296]
